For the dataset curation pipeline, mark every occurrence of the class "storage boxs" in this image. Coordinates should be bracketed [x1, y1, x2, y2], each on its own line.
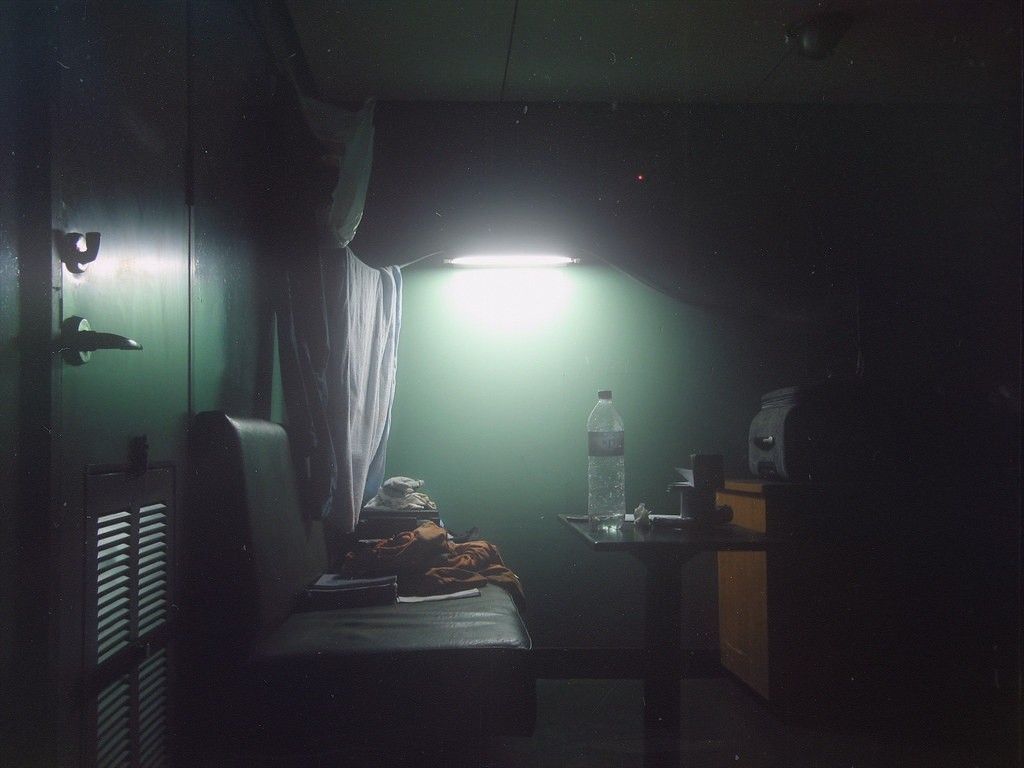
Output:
[359, 509, 438, 541]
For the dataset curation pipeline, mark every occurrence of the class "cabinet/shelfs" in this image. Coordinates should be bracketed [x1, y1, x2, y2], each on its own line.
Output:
[715, 478, 898, 711]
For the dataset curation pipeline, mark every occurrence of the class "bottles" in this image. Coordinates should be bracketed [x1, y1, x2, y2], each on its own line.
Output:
[586, 390, 626, 531]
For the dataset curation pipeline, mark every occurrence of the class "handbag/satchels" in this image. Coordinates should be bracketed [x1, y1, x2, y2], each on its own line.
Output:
[307, 574, 398, 611]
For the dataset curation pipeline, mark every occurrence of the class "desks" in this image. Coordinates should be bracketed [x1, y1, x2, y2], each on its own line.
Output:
[555, 514, 791, 768]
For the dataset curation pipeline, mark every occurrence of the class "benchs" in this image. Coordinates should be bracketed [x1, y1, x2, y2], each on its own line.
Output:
[194, 411, 538, 768]
